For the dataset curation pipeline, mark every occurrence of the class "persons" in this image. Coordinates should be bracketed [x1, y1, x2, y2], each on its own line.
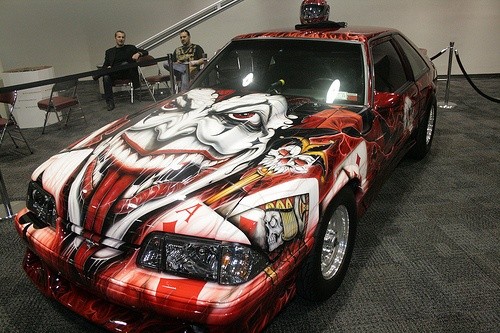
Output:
[163, 29, 207, 91]
[91, 30, 149, 111]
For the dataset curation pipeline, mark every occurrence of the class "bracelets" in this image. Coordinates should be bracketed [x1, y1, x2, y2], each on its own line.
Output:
[138, 52, 142, 56]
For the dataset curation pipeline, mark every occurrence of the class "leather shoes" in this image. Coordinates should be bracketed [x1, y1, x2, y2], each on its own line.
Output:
[93, 67, 110, 81]
[106, 101, 115, 110]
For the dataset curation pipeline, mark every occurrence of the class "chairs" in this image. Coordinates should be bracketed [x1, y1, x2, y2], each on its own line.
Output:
[37, 77, 88, 133]
[111, 79, 134, 103]
[0, 89, 34, 156]
[321, 46, 363, 99]
[135, 55, 172, 104]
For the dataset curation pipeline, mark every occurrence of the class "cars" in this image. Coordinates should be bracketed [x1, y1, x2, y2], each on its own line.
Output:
[13, 27, 439, 333]
